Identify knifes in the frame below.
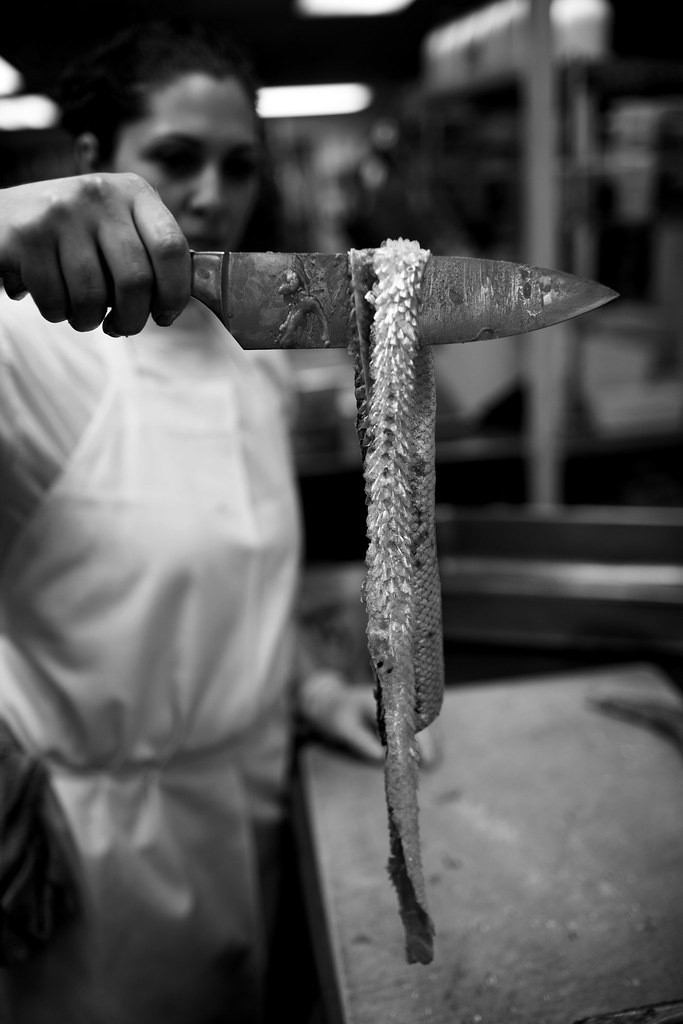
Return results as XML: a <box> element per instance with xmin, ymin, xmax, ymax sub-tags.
<box><xmin>1</xmin><ymin>249</ymin><xmax>622</xmax><ymax>350</ymax></box>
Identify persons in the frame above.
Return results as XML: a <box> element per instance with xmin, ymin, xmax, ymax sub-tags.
<box><xmin>0</xmin><ymin>28</ymin><xmax>443</xmax><ymax>1024</ymax></box>
